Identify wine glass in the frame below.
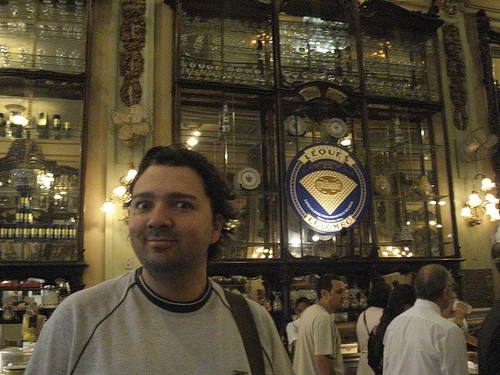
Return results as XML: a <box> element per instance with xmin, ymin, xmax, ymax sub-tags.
<box><xmin>180</xmin><ymin>60</ymin><xmax>423</xmax><ymax>95</ymax></box>
<box><xmin>0</xmin><ymin>0</ymin><xmax>84</xmax><ymax>72</ymax></box>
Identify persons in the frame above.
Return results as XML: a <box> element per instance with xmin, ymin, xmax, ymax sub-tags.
<box><xmin>491</xmin><ymin>226</ymin><xmax>500</xmax><ymax>273</ymax></box>
<box><xmin>286</xmin><ymin>296</ymin><xmax>310</xmax><ymax>356</ymax></box>
<box><xmin>353</xmin><ymin>282</ymin><xmax>393</xmax><ymax>375</ymax></box>
<box><xmin>291</xmin><ymin>274</ymin><xmax>346</xmax><ymax>375</ymax></box>
<box><xmin>365</xmin><ymin>283</ymin><xmax>417</xmax><ymax>375</ymax></box>
<box><xmin>438</xmin><ymin>283</ymin><xmax>469</xmax><ymax>334</ymax></box>
<box><xmin>23</xmin><ymin>145</ymin><xmax>296</xmax><ymax>375</ymax></box>
<box><xmin>382</xmin><ymin>264</ymin><xmax>469</xmax><ymax>375</ymax></box>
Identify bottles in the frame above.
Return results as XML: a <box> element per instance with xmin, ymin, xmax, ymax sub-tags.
<box><xmin>0</xmin><ymin>111</ymin><xmax>71</xmax><ymax>140</ymax></box>
<box><xmin>0</xmin><ymin>175</ymin><xmax>80</xmax><ymax>240</ymax></box>
<box><xmin>23</xmin><ymin>315</ymin><xmax>41</xmax><ymax>356</ymax></box>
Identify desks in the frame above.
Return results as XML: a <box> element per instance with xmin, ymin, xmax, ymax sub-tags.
<box><xmin>0</xmin><ymin>346</ymin><xmax>34</xmax><ymax>375</ymax></box>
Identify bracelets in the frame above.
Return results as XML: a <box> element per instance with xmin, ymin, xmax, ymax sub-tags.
<box><xmin>455</xmin><ymin>322</ymin><xmax>463</xmax><ymax>328</ymax></box>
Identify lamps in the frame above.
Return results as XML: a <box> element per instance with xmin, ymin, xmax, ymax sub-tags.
<box><xmin>461</xmin><ymin>173</ymin><xmax>500</xmax><ymax>228</ymax></box>
<box><xmin>100</xmin><ymin>163</ymin><xmax>137</xmax><ymax>227</ymax></box>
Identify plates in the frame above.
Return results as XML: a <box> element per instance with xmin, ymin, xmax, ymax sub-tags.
<box><xmin>3</xmin><ymin>362</ymin><xmax>28</xmax><ymax>372</ymax></box>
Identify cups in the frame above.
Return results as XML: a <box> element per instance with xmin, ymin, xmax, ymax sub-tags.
<box><xmin>453</xmin><ymin>301</ymin><xmax>472</xmax><ymax>314</ymax></box>
